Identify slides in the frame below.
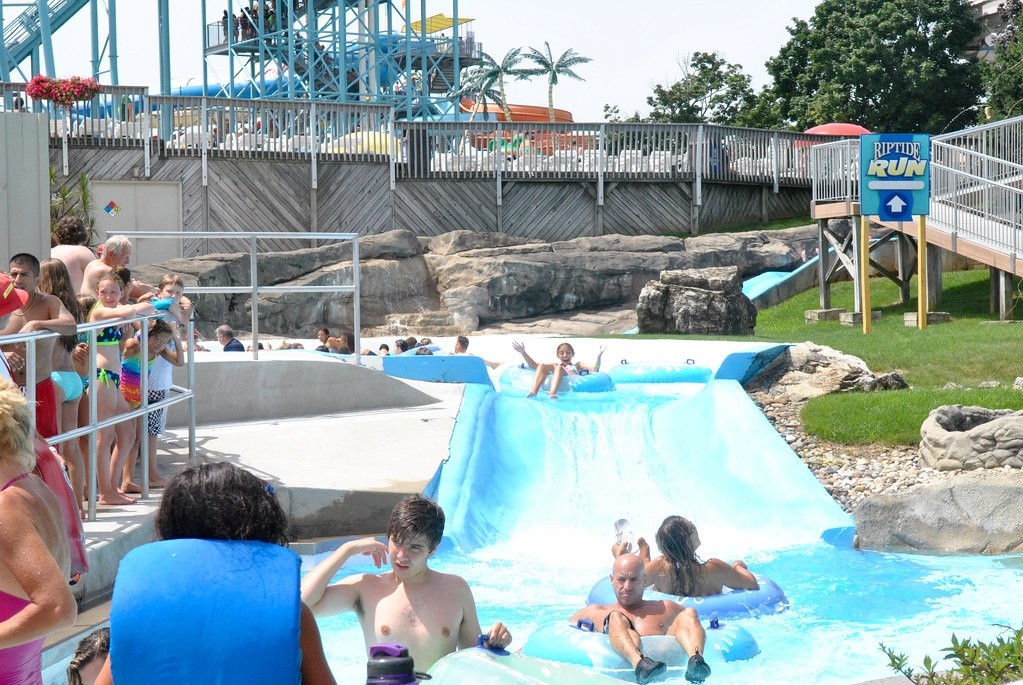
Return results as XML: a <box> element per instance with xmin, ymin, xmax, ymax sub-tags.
<box><xmin>423</xmin><ymin>378</ymin><xmax>862</xmax><ymax>554</ymax></box>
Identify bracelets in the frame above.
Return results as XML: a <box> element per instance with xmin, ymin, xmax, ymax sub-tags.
<box><xmin>734</xmin><ymin>564</ymin><xmax>744</xmax><ymax>567</ymax></box>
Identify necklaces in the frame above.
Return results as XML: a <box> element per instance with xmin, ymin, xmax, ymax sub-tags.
<box><xmin>13</xmin><ymin>291</ymin><xmax>37</xmax><ymax>316</ymax></box>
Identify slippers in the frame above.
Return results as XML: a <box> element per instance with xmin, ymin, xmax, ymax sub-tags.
<box><xmin>615</xmin><ymin>519</ymin><xmax>639</xmax><ymax>554</ymax></box>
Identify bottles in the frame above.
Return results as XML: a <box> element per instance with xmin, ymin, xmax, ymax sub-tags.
<box><xmin>366</xmin><ymin>643</ymin><xmax>418</xmax><ymax>685</ymax></box>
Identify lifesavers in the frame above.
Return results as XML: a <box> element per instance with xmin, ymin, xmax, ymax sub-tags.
<box><xmin>610</xmin><ymin>359</ymin><xmax>712</xmax><ymax>382</ymax></box>
<box><xmin>586</xmin><ymin>573</ymin><xmax>790</xmax><ymax>619</ymax></box>
<box><xmin>499</xmin><ymin>364</ymin><xmax>616</xmax><ymax>393</ymax></box>
<box><xmin>520</xmin><ymin>617</ymin><xmax>762</xmax><ymax>673</ymax></box>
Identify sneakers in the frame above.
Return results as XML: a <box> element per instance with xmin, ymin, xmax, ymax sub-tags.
<box><xmin>634</xmin><ymin>654</ymin><xmax>667</xmax><ymax>684</ymax></box>
<box><xmin>684</xmin><ymin>646</ymin><xmax>711</xmax><ymax>685</ymax></box>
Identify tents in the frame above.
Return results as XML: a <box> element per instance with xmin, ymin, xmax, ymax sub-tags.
<box><xmin>401</xmin><ymin>13</ymin><xmax>476</xmax><ymax>38</ymax></box>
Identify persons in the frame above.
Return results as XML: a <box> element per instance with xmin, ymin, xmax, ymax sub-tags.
<box><xmin>213</xmin><ymin>0</ymin><xmax>464</xmax><ymax>148</ymax></box>
<box><xmin>298</xmin><ymin>492</ymin><xmax>513</xmax><ymax>674</ymax></box>
<box><xmin>91</xmin><ymin>460</ymin><xmax>339</xmax><ymax>685</ymax></box>
<box><xmin>65</xmin><ymin>628</ymin><xmax>111</xmax><ymax>685</ymax></box>
<box><xmin>1</xmin><ymin>372</ymin><xmax>80</xmax><ymax>685</ymax></box>
<box><xmin>0</xmin><ymin>218</ymin><xmax>499</xmax><ymax>685</ymax></box>
<box><xmin>572</xmin><ymin>142</ymin><xmax>585</xmax><ymax>158</ymax></box>
<box><xmin>12</xmin><ymin>97</ymin><xmax>31</xmax><ymax>113</ymax></box>
<box><xmin>512</xmin><ymin>341</ymin><xmax>608</xmax><ymax>398</ymax></box>
<box><xmin>570</xmin><ymin>554</ymin><xmax>710</xmax><ymax>685</ymax></box>
<box><xmin>519</xmin><ymin>131</ymin><xmax>537</xmax><ymax>157</ymax></box>
<box><xmin>611</xmin><ymin>515</ymin><xmax>758</xmax><ymax>598</ymax></box>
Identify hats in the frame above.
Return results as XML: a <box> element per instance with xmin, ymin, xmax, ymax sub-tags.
<box><xmin>185</xmin><ymin>329</ymin><xmax>198</xmax><ymax>339</ymax></box>
